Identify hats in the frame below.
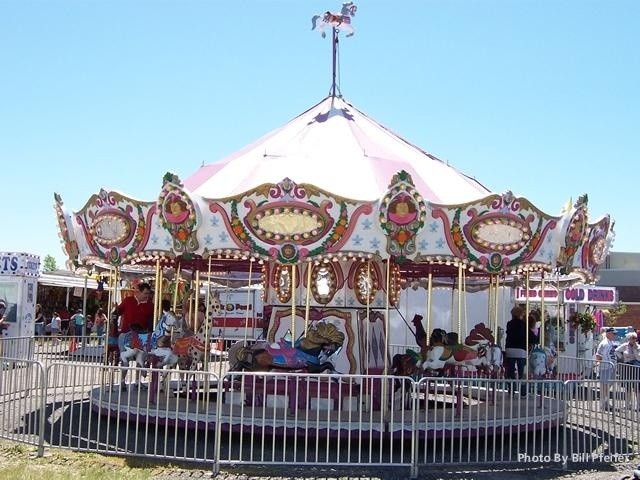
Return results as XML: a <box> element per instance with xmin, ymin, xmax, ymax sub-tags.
<box><xmin>606</xmin><ymin>327</ymin><xmax>619</xmax><ymax>335</ymax></box>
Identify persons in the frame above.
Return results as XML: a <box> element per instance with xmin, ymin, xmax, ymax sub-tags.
<box><xmin>505</xmin><ymin>306</ymin><xmax>551</xmax><ymax>399</ymax></box>
<box><xmin>35</xmin><ymin>304</ymin><xmax>108</xmax><ymax>346</ymax></box>
<box><xmin>0</xmin><ymin>298</ymin><xmax>10</xmax><ymax>357</ymax></box>
<box><xmin>596</xmin><ymin>328</ymin><xmax>618</xmax><ymax>411</ymax></box>
<box><xmin>114</xmin><ymin>282</ymin><xmax>155</xmax><ymax>391</ymax></box>
<box><xmin>615</xmin><ymin>330</ymin><xmax>640</xmax><ymax>411</ymax></box>
<box><xmin>198</xmin><ymin>303</ymin><xmax>207</xmax><ymax>313</ymax></box>
<box><xmin>185</xmin><ymin>300</ymin><xmax>204</xmax><ymax>331</ymax></box>
